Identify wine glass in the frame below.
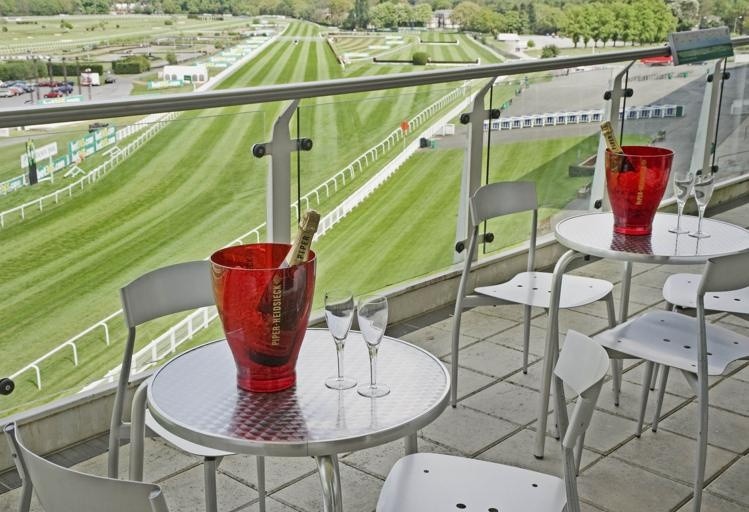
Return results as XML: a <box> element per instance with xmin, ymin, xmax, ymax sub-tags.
<box><xmin>355</xmin><ymin>295</ymin><xmax>391</xmax><ymax>399</ymax></box>
<box><xmin>319</xmin><ymin>289</ymin><xmax>358</xmax><ymax>389</ymax></box>
<box><xmin>668</xmin><ymin>171</ymin><xmax>694</xmax><ymax>234</ymax></box>
<box><xmin>688</xmin><ymin>174</ymin><xmax>715</xmax><ymax>238</ymax></box>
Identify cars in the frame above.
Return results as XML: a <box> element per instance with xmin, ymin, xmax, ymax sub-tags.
<box><xmin>0</xmin><ymin>76</ymin><xmax>75</xmax><ymax>100</ymax></box>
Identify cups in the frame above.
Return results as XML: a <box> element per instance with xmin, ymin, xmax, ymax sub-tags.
<box><xmin>227</xmin><ymin>386</ymin><xmax>316</xmax><ymax>445</ymax></box>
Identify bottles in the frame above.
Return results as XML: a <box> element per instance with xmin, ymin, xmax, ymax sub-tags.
<box><xmin>244</xmin><ymin>211</ymin><xmax>321</xmax><ymax>367</ymax></box>
<box><xmin>600</xmin><ymin>120</ymin><xmax>635</xmax><ymax>173</ymax></box>
